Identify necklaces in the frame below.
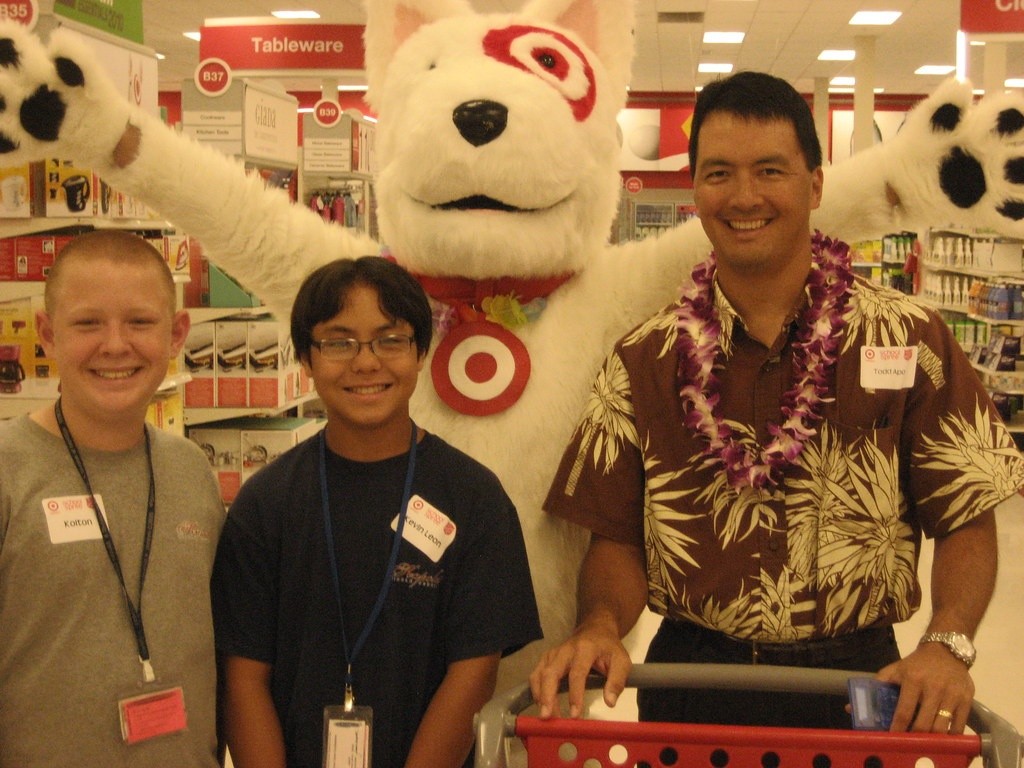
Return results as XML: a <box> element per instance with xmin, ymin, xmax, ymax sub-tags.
<box><xmin>676</xmin><ymin>227</ymin><xmax>854</xmax><ymax>495</ymax></box>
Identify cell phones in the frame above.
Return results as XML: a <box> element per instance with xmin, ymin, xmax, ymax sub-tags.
<box><xmin>847</xmin><ymin>676</ymin><xmax>921</xmax><ymax>733</ymax></box>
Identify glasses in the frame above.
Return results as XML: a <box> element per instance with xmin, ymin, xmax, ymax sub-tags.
<box><xmin>311</xmin><ymin>333</ymin><xmax>416</xmax><ymax>360</ymax></box>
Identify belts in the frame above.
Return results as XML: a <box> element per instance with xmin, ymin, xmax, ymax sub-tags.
<box><xmin>659</xmin><ymin>619</ymin><xmax>894</xmax><ymax>664</ymax></box>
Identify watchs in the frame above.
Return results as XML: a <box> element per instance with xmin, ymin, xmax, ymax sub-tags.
<box><xmin>919</xmin><ymin>632</ymin><xmax>977</xmax><ymax>671</ymax></box>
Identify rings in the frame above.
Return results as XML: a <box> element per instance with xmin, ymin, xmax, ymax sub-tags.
<box><xmin>937</xmin><ymin>710</ymin><xmax>953</xmax><ymax>720</ymax></box>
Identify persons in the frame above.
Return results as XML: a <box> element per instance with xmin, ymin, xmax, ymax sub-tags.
<box><xmin>0</xmin><ymin>230</ymin><xmax>229</xmax><ymax>768</ymax></box>
<box><xmin>214</xmin><ymin>255</ymin><xmax>519</xmax><ymax>768</ymax></box>
<box><xmin>532</xmin><ymin>73</ymin><xmax>999</xmax><ymax>735</ymax></box>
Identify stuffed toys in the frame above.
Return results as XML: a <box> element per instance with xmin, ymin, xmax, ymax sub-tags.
<box><xmin>0</xmin><ymin>0</ymin><xmax>1024</xmax><ymax>768</ymax></box>
